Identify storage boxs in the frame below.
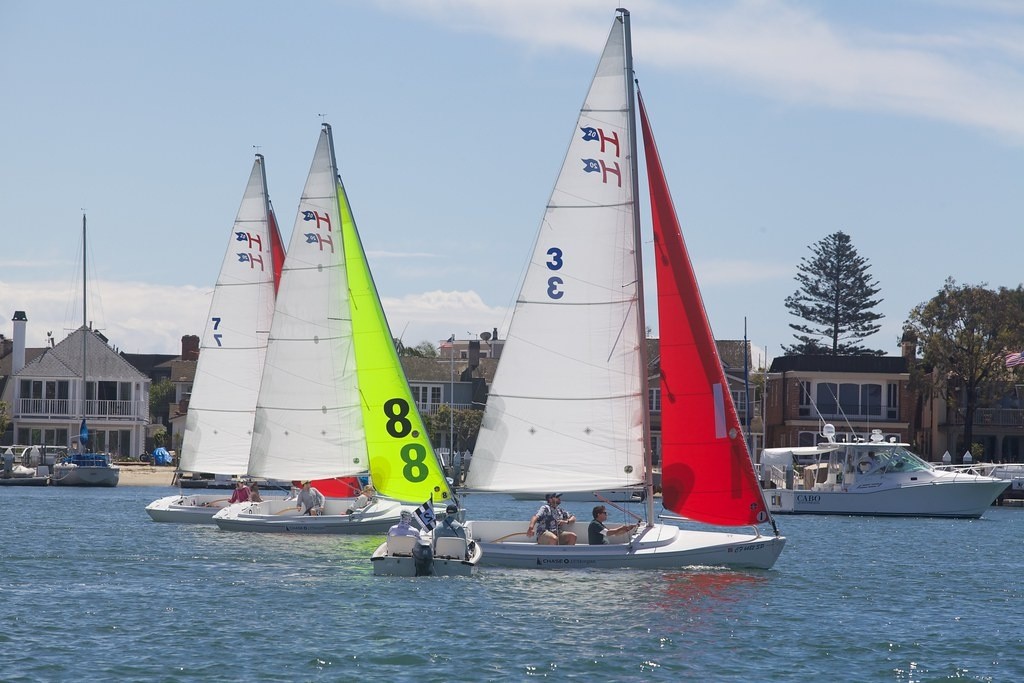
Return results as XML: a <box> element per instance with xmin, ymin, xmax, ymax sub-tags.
<box><xmin>803</xmin><ymin>463</ymin><xmax>839</xmax><ymax>490</ymax></box>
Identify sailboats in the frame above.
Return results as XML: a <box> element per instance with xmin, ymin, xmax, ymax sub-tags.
<box><xmin>52</xmin><ymin>214</ymin><xmax>119</xmax><ymax>487</ymax></box>
<box><xmin>335</xmin><ymin>174</ymin><xmax>484</xmax><ymax>577</ymax></box>
<box><xmin>145</xmin><ymin>154</ymin><xmax>372</xmax><ymax>525</ymax></box>
<box><xmin>461</xmin><ymin>6</ymin><xmax>788</xmax><ymax>570</ymax></box>
<box><xmin>212</xmin><ymin>123</ymin><xmax>467</xmax><ymax>534</ymax></box>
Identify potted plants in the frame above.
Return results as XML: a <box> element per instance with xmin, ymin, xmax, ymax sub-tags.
<box><xmin>149</xmin><ymin>377</ymin><xmax>176</xmax><ymax>423</ymax></box>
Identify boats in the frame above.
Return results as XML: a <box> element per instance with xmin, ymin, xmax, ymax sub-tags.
<box><xmin>761</xmin><ymin>424</ymin><xmax>1024</xmax><ymax>520</ymax></box>
<box><xmin>512</xmin><ymin>485</ymin><xmax>645</xmax><ymax>503</ymax></box>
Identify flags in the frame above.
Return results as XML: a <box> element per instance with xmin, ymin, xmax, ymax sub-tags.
<box><xmin>1005</xmin><ymin>351</ymin><xmax>1024</xmax><ymax>367</ymax></box>
<box><xmin>414</xmin><ymin>499</ymin><xmax>436</xmax><ymax>533</ymax></box>
<box><xmin>438</xmin><ymin>337</ymin><xmax>455</xmax><ymax>348</ymax></box>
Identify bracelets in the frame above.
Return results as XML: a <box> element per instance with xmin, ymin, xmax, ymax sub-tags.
<box><xmin>567</xmin><ymin>520</ymin><xmax>569</xmax><ymax>524</ymax></box>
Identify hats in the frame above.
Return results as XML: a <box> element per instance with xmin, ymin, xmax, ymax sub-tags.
<box><xmin>447</xmin><ymin>505</ymin><xmax>458</xmax><ymax>513</ymax></box>
<box><xmin>238</xmin><ymin>479</ymin><xmax>244</xmax><ymax>483</ymax></box>
<box><xmin>301</xmin><ymin>480</ymin><xmax>312</xmax><ymax>485</ymax></box>
<box><xmin>545</xmin><ymin>493</ymin><xmax>563</xmax><ymax>500</ymax></box>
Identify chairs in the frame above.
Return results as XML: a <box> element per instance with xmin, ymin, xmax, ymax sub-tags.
<box><xmin>388</xmin><ymin>535</ymin><xmax>417</xmax><ymax>557</ymax></box>
<box><xmin>436</xmin><ymin>536</ymin><xmax>465</xmax><ymax>560</ymax></box>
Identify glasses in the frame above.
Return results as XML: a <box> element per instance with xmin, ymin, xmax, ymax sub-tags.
<box><xmin>602</xmin><ymin>511</ymin><xmax>606</xmax><ymax>514</ymax></box>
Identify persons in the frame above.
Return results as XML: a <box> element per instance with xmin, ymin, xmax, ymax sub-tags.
<box><xmin>251</xmin><ymin>481</ymin><xmax>258</xmax><ymax>492</ymax></box>
<box><xmin>433</xmin><ymin>504</ymin><xmax>468</xmax><ymax>555</ymax></box>
<box><xmin>869</xmin><ymin>451</ymin><xmax>875</xmax><ymax>458</ymax></box>
<box><xmin>228</xmin><ymin>478</ymin><xmax>251</xmax><ymax>503</ymax></box>
<box><xmin>347</xmin><ymin>485</ymin><xmax>374</xmax><ymax>514</ymax></box>
<box><xmin>387</xmin><ymin>510</ymin><xmax>421</xmax><ymax>552</ymax></box>
<box><xmin>526</xmin><ymin>492</ymin><xmax>577</xmax><ymax>545</ymax></box>
<box><xmin>588</xmin><ymin>505</ymin><xmax>636</xmax><ymax>545</ymax></box>
<box><xmin>297</xmin><ymin>479</ymin><xmax>325</xmax><ymax>516</ymax></box>
